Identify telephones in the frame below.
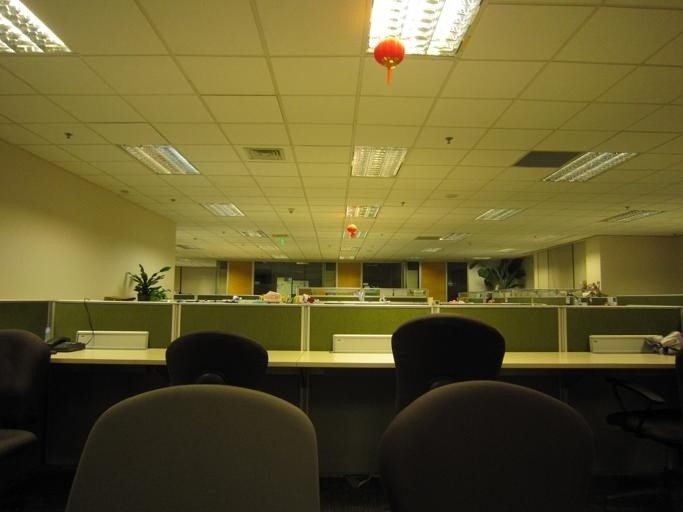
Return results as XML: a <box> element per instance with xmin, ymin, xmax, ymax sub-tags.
<box><xmin>45</xmin><ymin>336</ymin><xmax>86</xmax><ymax>352</ymax></box>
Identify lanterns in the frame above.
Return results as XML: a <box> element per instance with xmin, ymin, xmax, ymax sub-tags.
<box><xmin>372</xmin><ymin>34</ymin><xmax>406</xmax><ymax>85</ymax></box>
<box><xmin>347</xmin><ymin>224</ymin><xmax>357</xmax><ymax>239</ymax></box>
